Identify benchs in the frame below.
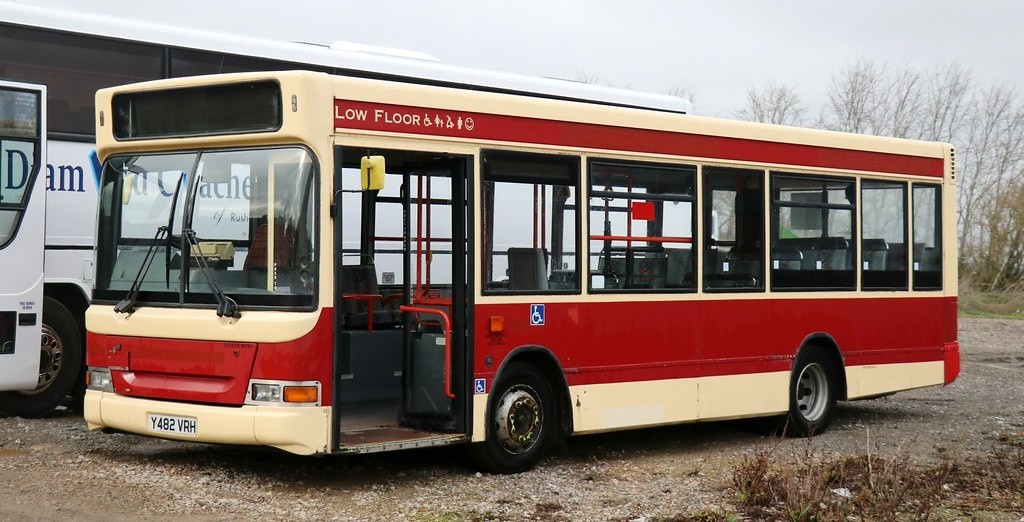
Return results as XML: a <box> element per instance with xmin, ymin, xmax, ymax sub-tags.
<box><xmin>597</xmin><ymin>237</ymin><xmax>925</xmax><ymax>288</ymax></box>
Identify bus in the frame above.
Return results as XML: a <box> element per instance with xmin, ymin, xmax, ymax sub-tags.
<box><xmin>81</xmin><ymin>69</ymin><xmax>964</xmax><ymax>474</ymax></box>
<box><xmin>0</xmin><ymin>79</ymin><xmax>725</xmax><ymax>422</ymax></box>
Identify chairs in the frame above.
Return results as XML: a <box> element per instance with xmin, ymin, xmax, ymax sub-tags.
<box><xmin>342</xmin><ymin>255</ymin><xmax>403</xmax><ymax>330</ymax></box>
<box><xmin>508</xmin><ymin>248</ymin><xmax>549</xmax><ymax>290</ymax></box>
